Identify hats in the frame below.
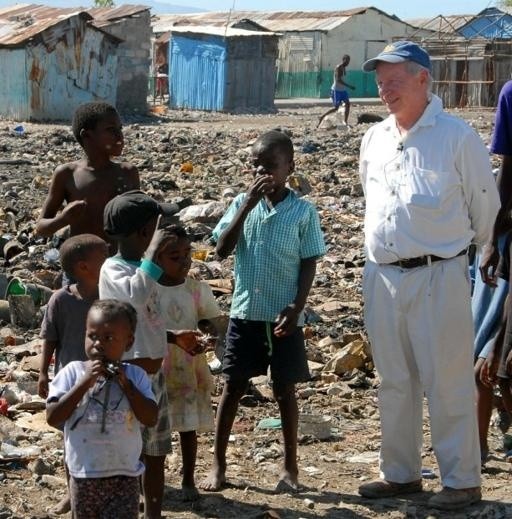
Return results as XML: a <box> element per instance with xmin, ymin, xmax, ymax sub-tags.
<box><xmin>362</xmin><ymin>41</ymin><xmax>432</xmax><ymax>72</ymax></box>
<box><xmin>104</xmin><ymin>190</ymin><xmax>179</xmax><ymax>237</ymax></box>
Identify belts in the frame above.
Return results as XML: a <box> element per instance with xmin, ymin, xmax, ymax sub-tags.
<box><xmin>388</xmin><ymin>249</ymin><xmax>467</xmax><ymax>268</ymax></box>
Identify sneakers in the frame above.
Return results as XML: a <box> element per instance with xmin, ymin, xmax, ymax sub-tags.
<box><xmin>427</xmin><ymin>488</ymin><xmax>481</xmax><ymax>509</ymax></box>
<box><xmin>359</xmin><ymin>479</ymin><xmax>422</xmax><ymax>498</ymax></box>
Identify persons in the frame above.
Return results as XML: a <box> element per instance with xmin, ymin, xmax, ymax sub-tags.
<box><xmin>194</xmin><ymin>131</ymin><xmax>326</xmax><ymax>492</ymax></box>
<box><xmin>46</xmin><ymin>300</ymin><xmax>158</xmax><ymax>519</ymax></box>
<box><xmin>35</xmin><ymin>103</ymin><xmax>143</xmax><ymax>257</ymax></box>
<box><xmin>97</xmin><ymin>189</ymin><xmax>214</xmax><ymax>519</ymax></box>
<box><xmin>152</xmin><ymin>224</ymin><xmax>222</xmax><ymax>501</ymax></box>
<box><xmin>38</xmin><ymin>234</ymin><xmax>109</xmax><ymax>514</ymax></box>
<box><xmin>316</xmin><ymin>54</ymin><xmax>356</xmax><ymax>130</ymax></box>
<box><xmin>472</xmin><ymin>82</ymin><xmax>512</xmax><ymax>477</ymax></box>
<box><xmin>359</xmin><ymin>40</ymin><xmax>502</xmax><ymax>512</ymax></box>
